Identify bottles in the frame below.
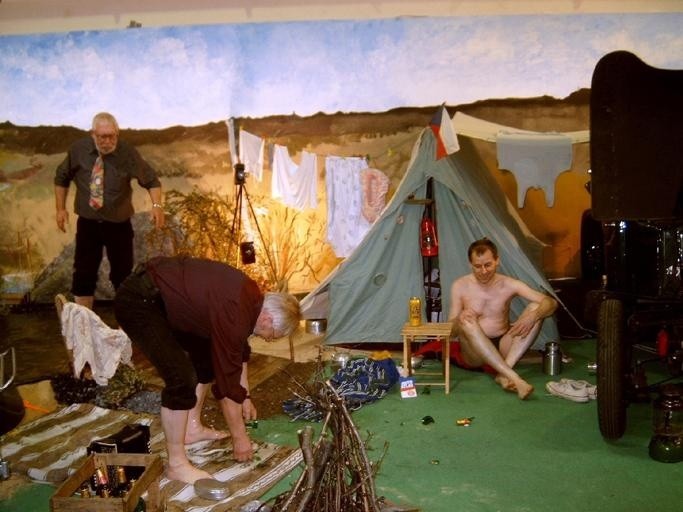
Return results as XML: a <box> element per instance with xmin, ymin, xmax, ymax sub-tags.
<box><xmin>586</xmin><ymin>362</ymin><xmax>596</xmax><ymax>375</ymax></box>
<box><xmin>79</xmin><ymin>466</ymin><xmax>145</xmax><ymax>512</ymax></box>
<box><xmin>408</xmin><ymin>296</ymin><xmax>421</xmax><ymax>326</ymax></box>
<box><xmin>542</xmin><ymin>342</ymin><xmax>561</xmax><ymax>376</ymax></box>
<box><xmin>599</xmin><ymin>275</ymin><xmax>608</xmax><ymax>299</ymax></box>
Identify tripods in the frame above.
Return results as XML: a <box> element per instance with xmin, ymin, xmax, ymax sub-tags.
<box><xmin>225</xmin><ymin>184</ymin><xmax>284</xmax><ymax>290</ymax></box>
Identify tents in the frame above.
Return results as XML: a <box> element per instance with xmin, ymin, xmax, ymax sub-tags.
<box><xmin>299</xmin><ymin>126</ymin><xmax>597</xmax><ymax>350</ymax></box>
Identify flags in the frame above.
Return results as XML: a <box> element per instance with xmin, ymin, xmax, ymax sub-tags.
<box><xmin>427</xmin><ymin>103</ymin><xmax>461</xmax><ymax>161</ymax></box>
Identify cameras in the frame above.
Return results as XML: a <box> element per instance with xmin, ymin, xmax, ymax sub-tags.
<box><xmin>234</xmin><ymin>163</ymin><xmax>246</xmax><ymax>185</ymax></box>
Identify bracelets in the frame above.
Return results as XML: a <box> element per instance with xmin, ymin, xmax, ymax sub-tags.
<box><xmin>152</xmin><ymin>203</ymin><xmax>162</xmax><ymax>209</ymax></box>
<box><xmin>244</xmin><ymin>396</ymin><xmax>250</xmax><ymax>399</ymax></box>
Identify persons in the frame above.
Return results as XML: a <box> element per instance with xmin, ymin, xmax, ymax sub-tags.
<box><xmin>53</xmin><ymin>112</ymin><xmax>166</xmax><ymax>311</ymax></box>
<box><xmin>447</xmin><ymin>240</ymin><xmax>558</xmax><ymax>399</ymax></box>
<box><xmin>113</xmin><ymin>256</ymin><xmax>301</xmax><ymax>487</ymax></box>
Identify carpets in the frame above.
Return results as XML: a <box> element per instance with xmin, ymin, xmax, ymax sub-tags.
<box><xmin>1</xmin><ymin>400</ymin><xmax>324</xmax><ymax>511</ymax></box>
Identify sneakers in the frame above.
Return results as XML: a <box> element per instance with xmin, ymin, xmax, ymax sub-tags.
<box><xmin>560</xmin><ymin>378</ymin><xmax>598</xmax><ymax>400</ymax></box>
<box><xmin>545</xmin><ymin>380</ymin><xmax>589</xmax><ymax>402</ymax></box>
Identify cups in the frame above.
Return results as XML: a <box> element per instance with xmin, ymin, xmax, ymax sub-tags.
<box><xmin>239</xmin><ymin>241</ymin><xmax>255</xmax><ymax>265</ymax></box>
<box><xmin>306</xmin><ymin>319</ymin><xmax>326</xmax><ymax>334</ymax></box>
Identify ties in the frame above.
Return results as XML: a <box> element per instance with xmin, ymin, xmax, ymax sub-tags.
<box><xmin>88</xmin><ymin>152</ymin><xmax>104</xmax><ymax>212</ymax></box>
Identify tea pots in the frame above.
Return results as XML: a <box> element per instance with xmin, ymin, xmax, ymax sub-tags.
<box><xmin>329</xmin><ymin>345</ymin><xmax>351</xmax><ymax>371</ymax></box>
<box><xmin>400</xmin><ymin>354</ymin><xmax>424</xmax><ymax>369</ymax></box>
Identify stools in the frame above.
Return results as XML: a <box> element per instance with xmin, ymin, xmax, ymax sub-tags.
<box><xmin>397</xmin><ymin>317</ymin><xmax>453</xmax><ymax>396</ymax></box>
